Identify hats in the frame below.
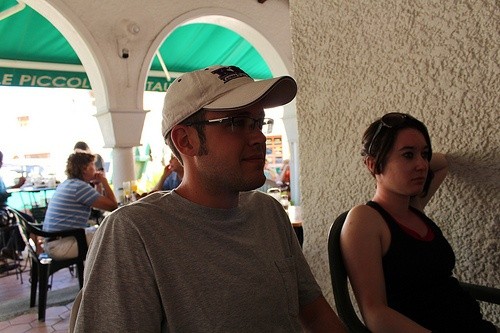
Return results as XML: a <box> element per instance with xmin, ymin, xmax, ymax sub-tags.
<box><xmin>162</xmin><ymin>66</ymin><xmax>297</xmax><ymax>140</ymax></box>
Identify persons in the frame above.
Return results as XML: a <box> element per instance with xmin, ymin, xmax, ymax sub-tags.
<box><xmin>41</xmin><ymin>151</ymin><xmax>118</xmax><ymax>261</ymax></box>
<box><xmin>68</xmin><ymin>64</ymin><xmax>349</xmax><ymax>333</ymax></box>
<box><xmin>151</xmin><ymin>150</ymin><xmax>185</xmax><ymax>195</ymax></box>
<box><xmin>0</xmin><ymin>150</ymin><xmax>46</xmax><ymax>261</ymax></box>
<box><xmin>74</xmin><ymin>140</ymin><xmax>106</xmax><ymax>228</ymax></box>
<box><xmin>340</xmin><ymin>111</ymin><xmax>499</xmax><ymax>332</ymax></box>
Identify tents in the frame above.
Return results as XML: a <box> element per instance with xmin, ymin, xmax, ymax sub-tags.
<box><xmin>0</xmin><ymin>0</ymin><xmax>280</xmax><ymax>94</ymax></box>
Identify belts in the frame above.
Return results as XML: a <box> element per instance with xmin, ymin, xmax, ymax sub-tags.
<box><xmin>46</xmin><ymin>236</ymin><xmax>67</xmax><ymax>243</ymax></box>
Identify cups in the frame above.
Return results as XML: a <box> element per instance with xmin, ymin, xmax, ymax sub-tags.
<box><xmin>288</xmin><ymin>206</ymin><xmax>295</xmax><ymax>221</ymax></box>
<box><xmin>48</xmin><ymin>174</ymin><xmax>56</xmax><ymax>186</ymax></box>
<box><xmin>132</xmin><ymin>181</ymin><xmax>138</xmax><ymax>192</ymax></box>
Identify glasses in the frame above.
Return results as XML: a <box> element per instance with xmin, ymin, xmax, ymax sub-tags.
<box><xmin>368</xmin><ymin>112</ymin><xmax>407</xmax><ymax>155</ymax></box>
<box><xmin>177</xmin><ymin>114</ymin><xmax>274</xmax><ymax>136</ymax></box>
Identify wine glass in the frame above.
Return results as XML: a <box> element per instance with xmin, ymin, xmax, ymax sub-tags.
<box><xmin>123</xmin><ymin>182</ymin><xmax>131</xmax><ymax>205</ymax></box>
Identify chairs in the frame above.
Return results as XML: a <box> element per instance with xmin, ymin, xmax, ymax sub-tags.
<box><xmin>0</xmin><ymin>207</ymin><xmax>89</xmax><ymax>321</ymax></box>
<box><xmin>328</xmin><ymin>210</ymin><xmax>500</xmax><ymax>333</ymax></box>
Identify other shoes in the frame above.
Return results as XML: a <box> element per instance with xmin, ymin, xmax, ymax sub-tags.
<box><xmin>0</xmin><ymin>247</ymin><xmax>24</xmax><ymax>261</ymax></box>
<box><xmin>0</xmin><ymin>263</ymin><xmax>20</xmax><ymax>274</ymax></box>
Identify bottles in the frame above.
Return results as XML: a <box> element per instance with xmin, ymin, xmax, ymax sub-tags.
<box><xmin>280</xmin><ymin>195</ymin><xmax>289</xmax><ymax>211</ymax></box>
